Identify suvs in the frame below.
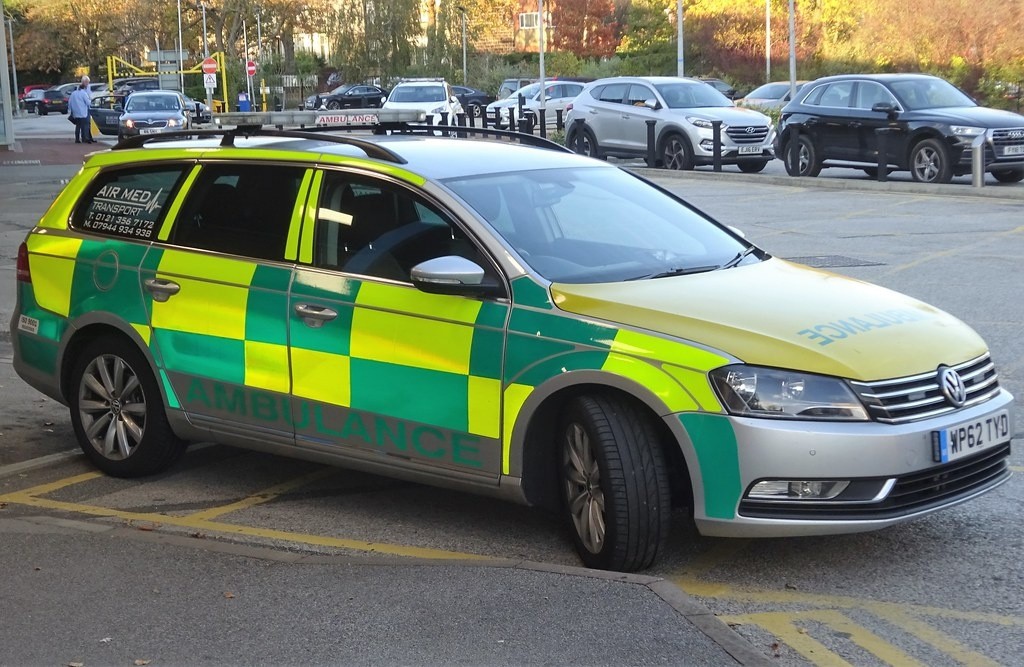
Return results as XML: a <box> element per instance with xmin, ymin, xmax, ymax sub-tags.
<box><xmin>9</xmin><ymin>126</ymin><xmax>1016</xmax><ymax>575</ymax></box>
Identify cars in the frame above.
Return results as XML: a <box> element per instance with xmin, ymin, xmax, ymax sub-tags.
<box><xmin>177</xmin><ymin>90</ymin><xmax>212</xmax><ymax>124</ymax></box>
<box><xmin>774</xmin><ymin>74</ymin><xmax>1024</xmax><ymax>183</ymax></box>
<box><xmin>114</xmin><ymin>89</ymin><xmax>191</xmax><ymax>144</ymax></box>
<box><xmin>47</xmin><ymin>82</ymin><xmax>106</xmax><ymax>99</ymax></box>
<box><xmin>95</xmin><ymin>76</ymin><xmax>160</xmax><ymax>91</ymax></box>
<box><xmin>563</xmin><ymin>76</ymin><xmax>778</xmax><ymax>174</ymax></box>
<box><xmin>87</xmin><ymin>97</ymin><xmax>125</xmax><ymax>132</ymax></box>
<box><xmin>698</xmin><ymin>77</ymin><xmax>745</xmax><ymax>101</ymax></box>
<box><xmin>304</xmin><ymin>84</ymin><xmax>390</xmax><ymax>110</ymax></box>
<box><xmin>18</xmin><ymin>88</ymin><xmax>69</xmax><ymax>115</ymax></box>
<box><xmin>451</xmin><ymin>86</ymin><xmax>496</xmax><ymax>118</ymax></box>
<box><xmin>19</xmin><ymin>85</ymin><xmax>52</xmax><ymax>99</ymax></box>
<box><xmin>734</xmin><ymin>81</ymin><xmax>814</xmax><ymax>117</ymax></box>
<box><xmin>485</xmin><ymin>81</ymin><xmax>588</xmax><ymax>129</ymax></box>
<box><xmin>378</xmin><ymin>77</ymin><xmax>465</xmax><ymax>141</ymax></box>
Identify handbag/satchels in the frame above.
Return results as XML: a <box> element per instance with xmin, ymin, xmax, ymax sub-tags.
<box><xmin>68</xmin><ymin>115</ymin><xmax>80</xmax><ymax>125</ymax></box>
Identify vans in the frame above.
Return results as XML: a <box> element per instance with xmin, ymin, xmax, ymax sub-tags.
<box><xmin>495</xmin><ymin>78</ymin><xmax>539</xmax><ymax>100</ymax></box>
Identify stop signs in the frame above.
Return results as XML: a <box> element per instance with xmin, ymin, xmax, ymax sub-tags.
<box><xmin>246</xmin><ymin>62</ymin><xmax>256</xmax><ymax>77</ymax></box>
<box><xmin>202</xmin><ymin>58</ymin><xmax>217</xmax><ymax>74</ymax></box>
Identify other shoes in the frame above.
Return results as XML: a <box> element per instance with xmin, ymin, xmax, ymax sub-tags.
<box><xmin>75</xmin><ymin>140</ymin><xmax>81</xmax><ymax>143</ymax></box>
<box><xmin>85</xmin><ymin>141</ymin><xmax>91</xmax><ymax>144</ymax></box>
<box><xmin>91</xmin><ymin>138</ymin><xmax>97</xmax><ymax>142</ymax></box>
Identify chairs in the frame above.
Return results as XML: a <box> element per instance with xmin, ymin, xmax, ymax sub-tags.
<box><xmin>344</xmin><ymin>196</ymin><xmax>424</xmax><ymax>281</ymax></box>
<box><xmin>825</xmin><ymin>91</ymin><xmax>841</xmax><ymax>106</ymax></box>
<box><xmin>861</xmin><ymin>85</ymin><xmax>878</xmax><ymax>109</ymax></box>
<box><xmin>666</xmin><ymin>89</ymin><xmax>680</xmax><ymax>105</ymax></box>
<box><xmin>445</xmin><ymin>182</ymin><xmax>524</xmax><ymax>273</ymax></box>
<box><xmin>889</xmin><ymin>90</ymin><xmax>908</xmax><ymax>109</ymax></box>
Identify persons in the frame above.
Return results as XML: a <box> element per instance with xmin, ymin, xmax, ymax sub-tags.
<box><xmin>69</xmin><ymin>85</ymin><xmax>91</xmax><ymax>143</ymax></box>
<box><xmin>122</xmin><ymin>89</ymin><xmax>130</xmax><ymax>103</ymax></box>
<box><xmin>315</xmin><ymin>95</ymin><xmax>322</xmax><ymax>109</ymax></box>
<box><xmin>77</xmin><ymin>76</ymin><xmax>97</xmax><ymax>142</ymax></box>
